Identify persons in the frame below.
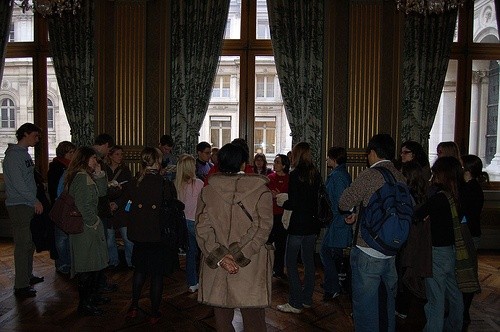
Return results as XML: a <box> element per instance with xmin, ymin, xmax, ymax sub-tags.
<box><xmin>460</xmin><ymin>154</ymin><xmax>483</xmax><ymax>332</ymax></box>
<box><xmin>203</xmin><ymin>139</ymin><xmax>253</xmax><ymax>188</ymax></box>
<box><xmin>319</xmin><ymin>146</ymin><xmax>352</xmax><ymax>301</ymax></box>
<box><xmin>64</xmin><ymin>147</ymin><xmax>110</xmax><ymax>318</ymax></box>
<box><xmin>95</xmin><ymin>135</ymin><xmax>114</xmax><ymax>155</ymax></box>
<box><xmin>337</xmin><ymin>134</ymin><xmax>412</xmax><ymax>332</ymax></box>
<box><xmin>48</xmin><ymin>141</ymin><xmax>76</xmax><ymax>276</ymax></box>
<box><xmin>113</xmin><ymin>146</ymin><xmax>177</xmax><ymax>324</ymax></box>
<box><xmin>264</xmin><ymin>154</ymin><xmax>301</xmax><ymax>262</ymax></box>
<box><xmin>412</xmin><ymin>155</ymin><xmax>475</xmax><ymax>332</ymax></box>
<box><xmin>394</xmin><ymin>141</ymin><xmax>432</xmax><ymax>319</ymax></box>
<box><xmin>437</xmin><ymin>142</ymin><xmax>461</xmax><ymax>160</ymax></box>
<box><xmin>173</xmin><ymin>154</ymin><xmax>205</xmax><ymax>293</ymax></box>
<box><xmin>3</xmin><ymin>122</ymin><xmax>45</xmax><ymax>297</ymax></box>
<box><xmin>194</xmin><ymin>143</ymin><xmax>274</xmax><ymax>332</ymax></box>
<box><xmin>98</xmin><ymin>146</ymin><xmax>134</xmax><ymax>270</ymax></box>
<box><xmin>254</xmin><ymin>153</ymin><xmax>273</xmax><ymax>176</ymax></box>
<box><xmin>287</xmin><ymin>151</ymin><xmax>294</xmax><ymax>169</ymax></box>
<box><xmin>276</xmin><ymin>142</ymin><xmax>327</xmax><ymax>314</ymax></box>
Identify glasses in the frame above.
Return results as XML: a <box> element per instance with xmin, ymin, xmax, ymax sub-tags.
<box><xmin>400</xmin><ymin>151</ymin><xmax>412</xmax><ymax>155</ymax></box>
<box><xmin>437</xmin><ymin>150</ymin><xmax>443</xmax><ymax>154</ymax></box>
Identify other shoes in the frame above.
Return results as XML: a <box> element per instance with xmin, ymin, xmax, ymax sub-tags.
<box><xmin>14</xmin><ymin>287</ymin><xmax>36</xmax><ymax>294</ymax></box>
<box><xmin>319</xmin><ymin>282</ymin><xmax>343</xmax><ymax>302</ymax></box>
<box><xmin>178</xmin><ymin>248</ymin><xmax>187</xmax><ymax>255</ymax></box>
<box><xmin>77</xmin><ymin>303</ymin><xmax>103</xmax><ymax>315</ymax></box>
<box><xmin>91</xmin><ymin>295</ymin><xmax>113</xmax><ymax>305</ymax></box>
<box><xmin>56</xmin><ymin>265</ymin><xmax>71</xmax><ymax>273</ymax></box>
<box><xmin>189</xmin><ymin>282</ymin><xmax>199</xmax><ymax>292</ymax></box>
<box><xmin>127</xmin><ymin>308</ymin><xmax>137</xmax><ymax>317</ymax></box>
<box><xmin>150</xmin><ymin>316</ymin><xmax>161</xmax><ymax>324</ymax></box>
<box><xmin>30</xmin><ymin>275</ymin><xmax>44</xmax><ymax>282</ymax></box>
<box><xmin>97</xmin><ymin>284</ymin><xmax>117</xmax><ymax>293</ymax></box>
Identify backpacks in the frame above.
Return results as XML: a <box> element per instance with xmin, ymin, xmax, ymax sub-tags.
<box><xmin>353</xmin><ymin>166</ymin><xmax>414</xmax><ymax>256</ymax></box>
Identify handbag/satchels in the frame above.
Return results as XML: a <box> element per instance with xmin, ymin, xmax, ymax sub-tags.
<box><xmin>48</xmin><ymin>170</ymin><xmax>84</xmax><ymax>234</ymax></box>
<box><xmin>159</xmin><ymin>180</ymin><xmax>188</xmax><ymax>249</ymax></box>
<box><xmin>312</xmin><ymin>181</ymin><xmax>334</xmax><ymax>229</ymax></box>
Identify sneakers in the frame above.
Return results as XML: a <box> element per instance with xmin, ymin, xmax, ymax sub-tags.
<box><xmin>276</xmin><ymin>303</ymin><xmax>311</xmax><ymax>313</ymax></box>
